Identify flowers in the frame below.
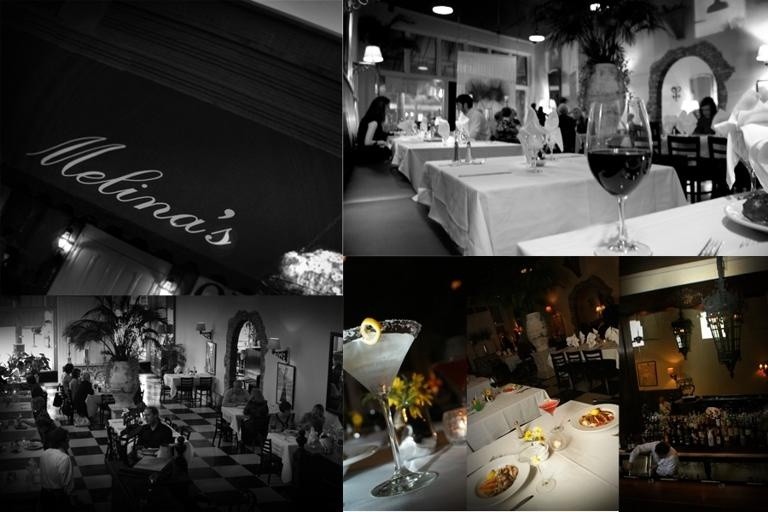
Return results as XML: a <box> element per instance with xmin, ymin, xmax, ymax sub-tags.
<box><xmin>361</xmin><ymin>372</ymin><xmax>444</xmax><ymax>422</ymax></box>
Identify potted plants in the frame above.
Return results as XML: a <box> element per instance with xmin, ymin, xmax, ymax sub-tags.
<box><xmin>530</xmin><ymin>0</ymin><xmax>682</xmax><ymax>154</ymax></box>
<box><xmin>61</xmin><ymin>295</ymin><xmax>172</xmax><ymax>406</ymax></box>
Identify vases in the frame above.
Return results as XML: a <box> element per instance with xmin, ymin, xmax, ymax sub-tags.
<box><xmin>407</xmin><ymin>405</ymin><xmax>441</xmax><ymax>450</ymax></box>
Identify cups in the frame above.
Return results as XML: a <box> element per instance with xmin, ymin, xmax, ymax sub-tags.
<box><xmin>549</xmin><ymin>434</ymin><xmax>566</xmax><ymax>450</ymax></box>
<box><xmin>442</xmin><ymin>407</ymin><xmax>467</xmax><ymax>444</ymax></box>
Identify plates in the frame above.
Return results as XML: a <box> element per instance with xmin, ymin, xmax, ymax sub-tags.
<box><xmin>722</xmin><ymin>194</ymin><xmax>768</xmax><ymax>232</ymax></box>
<box><xmin>502</xmin><ymin>383</ymin><xmax>521</xmax><ymax>395</ymax></box>
<box><xmin>569</xmin><ymin>403</ymin><xmax>618</xmax><ymax>433</ymax></box>
<box><xmin>343</xmin><ymin>444</ymin><xmax>376</xmax><ymax>467</ymax></box>
<box><xmin>466</xmin><ymin>453</ymin><xmax>529</xmax><ymax>509</ymax></box>
<box><xmin>23</xmin><ymin>442</ymin><xmax>43</xmax><ymax>450</ymax></box>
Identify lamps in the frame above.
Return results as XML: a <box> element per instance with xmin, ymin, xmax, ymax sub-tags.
<box><xmin>595</xmin><ymin>305</ymin><xmax>605</xmax><ymax>319</ymax></box>
<box><xmin>352</xmin><ymin>44</ymin><xmax>385</xmax><ymax>74</ymax></box>
<box><xmin>194</xmin><ymin>322</ymin><xmax>212</xmax><ymax>341</ymax></box>
<box><xmin>280</xmin><ymin>248</ymin><xmax>343</xmax><ymax>296</ymax></box>
<box><xmin>670</xmin><ymin>308</ymin><xmax>692</xmax><ymax>361</ymax></box>
<box><xmin>157</xmin><ymin>274</ymin><xmax>177</xmax><ymax>295</ymax></box>
<box><xmin>667</xmin><ymin>368</ymin><xmax>677</xmax><ymax>382</ymax></box>
<box><xmin>267</xmin><ymin>337</ymin><xmax>289</xmax><ymax>362</ymax></box>
<box><xmin>524</xmin><ymin>0</ymin><xmax>545</xmax><ymax>44</ymax></box>
<box><xmin>705</xmin><ymin>257</ymin><xmax>747</xmax><ymax>379</ymax></box>
<box><xmin>57</xmin><ymin>228</ymin><xmax>74</xmax><ymax>254</ymax></box>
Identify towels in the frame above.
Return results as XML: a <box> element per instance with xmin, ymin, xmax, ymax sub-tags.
<box><xmin>565</xmin><ymin>326</ymin><xmax>619</xmax><ymax>348</ymax></box>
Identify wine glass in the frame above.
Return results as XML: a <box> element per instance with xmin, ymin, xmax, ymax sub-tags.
<box><xmin>516</xmin><ymin>434</ymin><xmax>557</xmax><ymax>494</ymax></box>
<box><xmin>537</xmin><ymin>398</ymin><xmax>566</xmax><ymax>434</ymax></box>
<box><xmin>522</xmin><ymin>127</ymin><xmax>560</xmax><ymax>175</ymax></box>
<box><xmin>343</xmin><ymin>315</ymin><xmax>441</xmax><ymax>499</ymax></box>
<box><xmin>585</xmin><ymin>93</ymin><xmax>659</xmax><ymax>256</ymax></box>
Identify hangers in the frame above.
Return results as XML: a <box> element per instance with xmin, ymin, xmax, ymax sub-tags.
<box><xmin>343</xmin><ymin>420</ymin><xmax>467</xmax><ymax>511</ymax></box>
<box><xmin>466</xmin><ymin>336</ymin><xmax>619</xmax><ymax>511</ymax></box>
<box><xmin>386</xmin><ymin>123</ymin><xmax>767</xmax><ymax>256</ymax></box>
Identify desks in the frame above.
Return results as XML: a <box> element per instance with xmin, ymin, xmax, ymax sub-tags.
<box><xmin>620</xmin><ymin>478</ymin><xmax>768</xmax><ymax>512</ymax></box>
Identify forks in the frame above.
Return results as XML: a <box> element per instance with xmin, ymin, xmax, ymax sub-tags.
<box><xmin>697</xmin><ymin>236</ymin><xmax>725</xmax><ymax>257</ymax></box>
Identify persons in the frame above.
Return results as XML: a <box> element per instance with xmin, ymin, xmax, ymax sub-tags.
<box><xmin>692</xmin><ymin>96</ymin><xmax>719</xmax><ymax>133</ymax></box>
<box><xmin>624</xmin><ymin>439</ymin><xmax>681</xmax><ymax>476</ymax></box>
<box><xmin>453</xmin><ymin>96</ymin><xmax>492</xmax><ymax>140</ymax></box>
<box><xmin>136</xmin><ymin>405</ymin><xmax>173</xmax><ymax>459</ymax></box>
<box><xmin>221</xmin><ymin>380</ymin><xmax>248</xmax><ymax>407</ymax></box>
<box><xmin>241</xmin><ymin>387</ymin><xmax>270</xmax><ymax>439</ymax></box>
<box><xmin>37</xmin><ymin>428</ymin><xmax>80</xmax><ymax>511</ymax></box>
<box><xmin>356</xmin><ymin>96</ymin><xmax>393</xmax><ymax>169</ymax></box>
<box><xmin>0</xmin><ymin>359</ymin><xmax>96</xmax><ymax>438</ymax></box>
<box><xmin>302</xmin><ymin>403</ymin><xmax>324</xmax><ymax>435</ymax></box>
<box><xmin>497</xmin><ymin>103</ymin><xmax>587</xmax><ymax>152</ymax></box>
<box><xmin>273</xmin><ymin>401</ymin><xmax>298</xmax><ymax>431</ymax></box>
<box><xmin>277</xmin><ymin>367</ymin><xmax>288</xmax><ymax>402</ymax></box>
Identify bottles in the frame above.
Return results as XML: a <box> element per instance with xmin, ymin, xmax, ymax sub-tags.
<box><xmin>450</xmin><ymin>141</ymin><xmax>474</xmax><ymax>163</ymax></box>
<box><xmin>513</xmin><ymin>421</ymin><xmax>524</xmax><ymax>439</ymax></box>
<box><xmin>640</xmin><ymin>411</ymin><xmax>764</xmax><ymax>449</ymax></box>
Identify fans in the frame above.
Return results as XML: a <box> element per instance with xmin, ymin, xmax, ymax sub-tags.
<box><xmin>630</xmin><ymin>314</ymin><xmax>661</xmax><ymax>348</ymax></box>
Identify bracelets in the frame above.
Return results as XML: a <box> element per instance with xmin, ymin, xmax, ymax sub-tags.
<box><xmin>375</xmin><ymin>140</ymin><xmax>378</xmax><ymax>146</ymax></box>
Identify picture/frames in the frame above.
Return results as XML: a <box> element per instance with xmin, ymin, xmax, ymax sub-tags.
<box><xmin>205</xmin><ymin>340</ymin><xmax>217</xmax><ymax>375</ymax></box>
<box><xmin>324</xmin><ymin>331</ymin><xmax>343</xmax><ymax>418</ymax></box>
<box><xmin>637</xmin><ymin>361</ymin><xmax>659</xmax><ymax>387</ymax></box>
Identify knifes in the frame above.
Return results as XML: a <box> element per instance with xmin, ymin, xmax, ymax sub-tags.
<box><xmin>510</xmin><ymin>494</ymin><xmax>536</xmax><ymax>511</ymax></box>
